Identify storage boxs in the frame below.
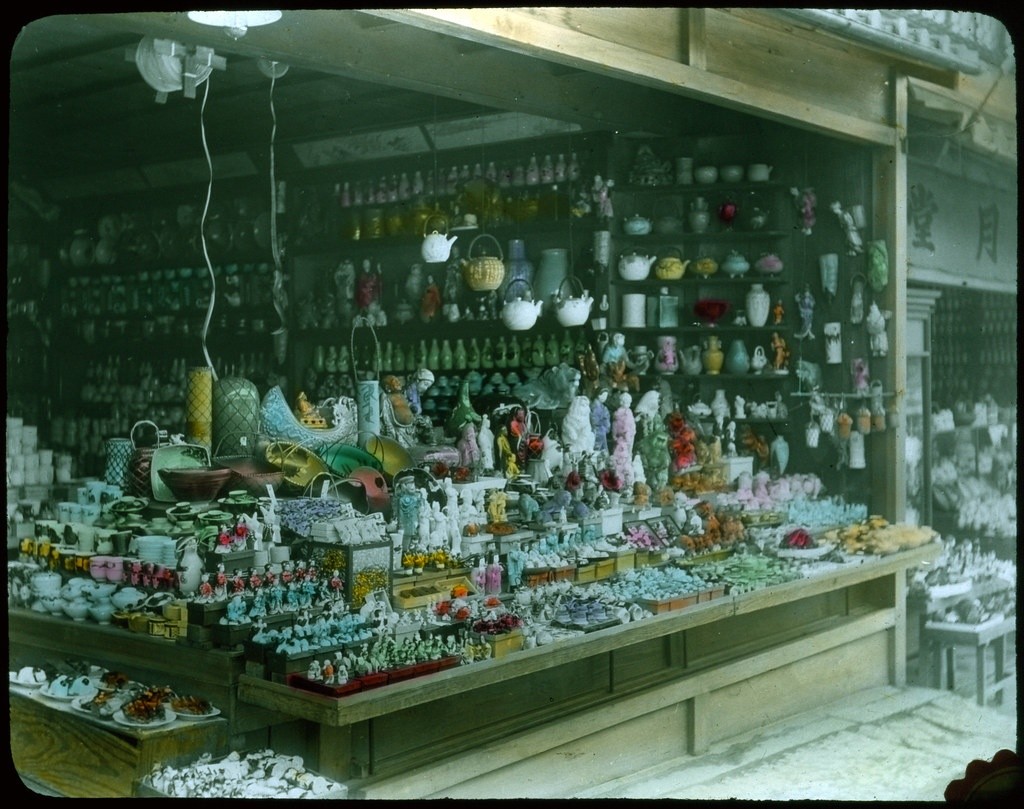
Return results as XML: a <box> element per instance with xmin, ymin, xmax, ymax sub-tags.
<box><xmin>189</xmin><ymin>489</ymin><xmax>786</xmax><ymax>698</ymax></box>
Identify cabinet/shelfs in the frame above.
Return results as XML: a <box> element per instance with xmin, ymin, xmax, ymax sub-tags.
<box><xmin>905</xmin><ymin>573</ymin><xmax>1016</xmax><ymax>706</ymax></box>
<box><xmin>54</xmin><ymin>177</ymin><xmax>790</xmax><ymax>459</ymax></box>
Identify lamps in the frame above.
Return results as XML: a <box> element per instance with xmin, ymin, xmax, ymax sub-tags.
<box><xmin>187</xmin><ymin>10</ymin><xmax>283</xmax><ymax>41</ymax></box>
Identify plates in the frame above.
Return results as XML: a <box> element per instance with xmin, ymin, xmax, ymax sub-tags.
<box><xmin>112</xmin><ymin>706</ymin><xmax>178</xmax><ymax>728</ymax></box>
<box><xmin>70</xmin><ymin>694</ymin><xmax>124</xmax><ymax>716</ymax></box>
<box><xmin>38</xmin><ymin>681</ymin><xmax>99</xmax><ymax>702</ymax></box>
<box><xmin>135</xmin><ymin>535</ymin><xmax>180</xmax><ymax>570</ymax></box>
<box><xmin>8</xmin><ymin>670</ymin><xmax>46</xmax><ymax>688</ymax></box>
<box><xmin>167</xmin><ymin>702</ymin><xmax>222</xmax><ymax>721</ymax></box>
<box><xmin>56</xmin><ymin>663</ymin><xmax>178</xmax><ymax>709</ymax></box>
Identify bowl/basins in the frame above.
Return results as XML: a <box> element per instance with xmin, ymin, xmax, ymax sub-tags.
<box><xmin>112</xmin><ymin>491</ymin><xmax>258</xmax><ymax>548</ymax></box>
<box><xmin>6</xmin><ymin>412</ymin><xmax>131</xmax><ymax>627</ymax></box>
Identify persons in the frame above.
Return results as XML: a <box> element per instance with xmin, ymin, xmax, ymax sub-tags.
<box><xmin>355</xmin><ymin>259</ymin><xmax>382</xmax><ymax>316</ymax></box>
<box><xmin>790</xmin><ymin>187</ymin><xmax>889</xmax><ymax>358</ymax></box>
<box><xmin>417</xmin><ymin>275</ymin><xmax>441</xmax><ymax>323</ymax></box>
<box><xmin>591</xmin><ymin>172</ymin><xmax>614</xmax><ymax>222</ymax></box>
<box><xmin>198</xmin><ymin>333</ymin><xmax>933</xmax><ymax>688</ymax></box>
